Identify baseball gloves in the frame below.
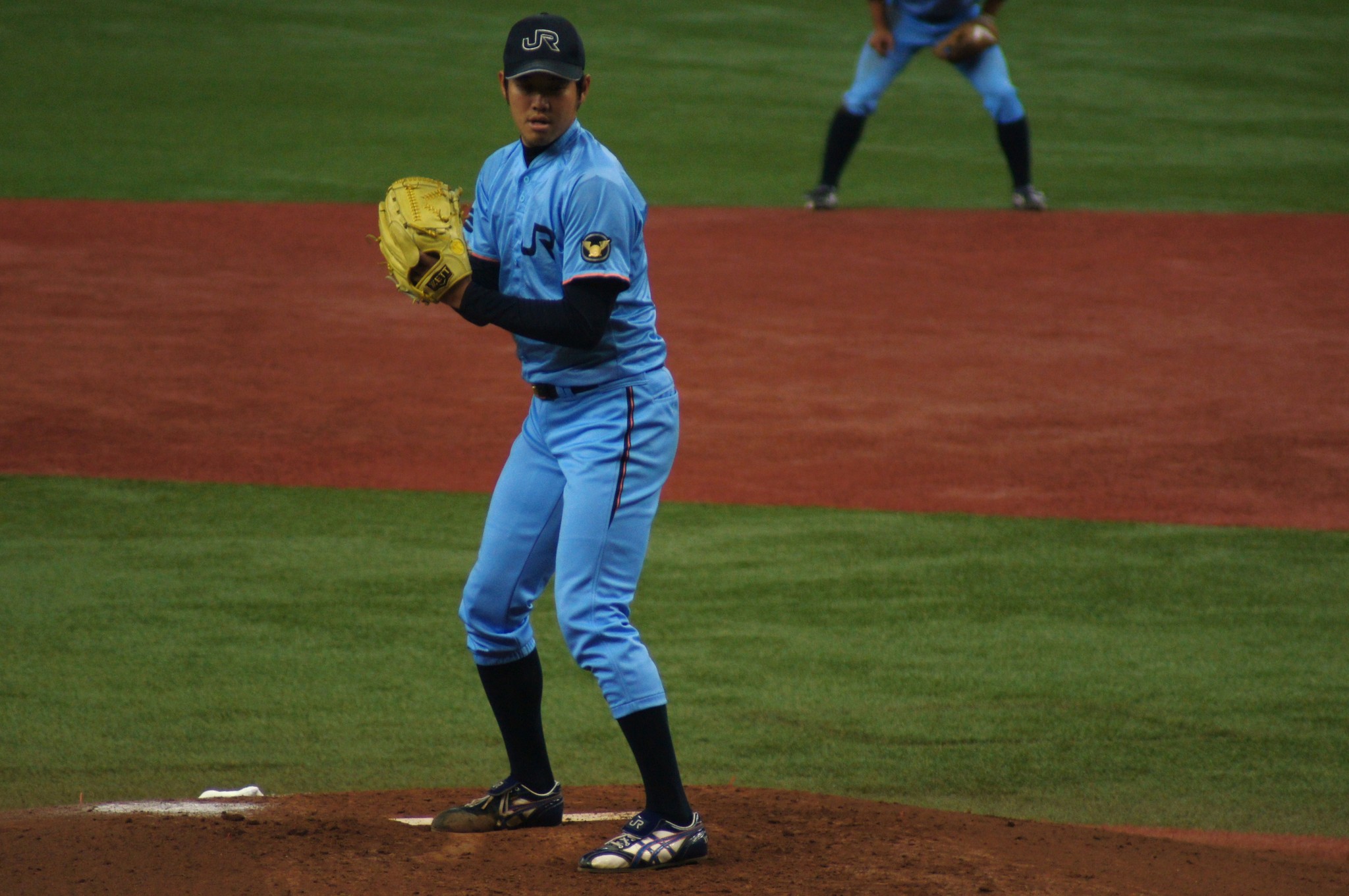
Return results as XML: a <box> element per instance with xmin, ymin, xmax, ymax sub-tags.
<box><xmin>934</xmin><ymin>14</ymin><xmax>998</xmax><ymax>64</ymax></box>
<box><xmin>377</xmin><ymin>176</ymin><xmax>475</xmax><ymax>305</ymax></box>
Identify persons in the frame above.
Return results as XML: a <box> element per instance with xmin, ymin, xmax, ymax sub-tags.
<box><xmin>399</xmin><ymin>11</ymin><xmax>721</xmax><ymax>873</ymax></box>
<box><xmin>801</xmin><ymin>0</ymin><xmax>1047</xmax><ymax>213</ymax></box>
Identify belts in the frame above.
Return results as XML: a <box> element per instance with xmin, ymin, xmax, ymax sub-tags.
<box><xmin>532</xmin><ymin>380</ymin><xmax>599</xmax><ymax>402</ymax></box>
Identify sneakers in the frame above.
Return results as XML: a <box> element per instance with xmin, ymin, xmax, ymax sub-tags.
<box><xmin>805</xmin><ymin>183</ymin><xmax>836</xmax><ymax>209</ymax></box>
<box><xmin>579</xmin><ymin>804</ymin><xmax>709</xmax><ymax>871</ymax></box>
<box><xmin>431</xmin><ymin>775</ymin><xmax>562</xmax><ymax>831</ymax></box>
<box><xmin>1012</xmin><ymin>184</ymin><xmax>1046</xmax><ymax>210</ymax></box>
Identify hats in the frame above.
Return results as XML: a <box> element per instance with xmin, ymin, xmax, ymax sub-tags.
<box><xmin>502</xmin><ymin>10</ymin><xmax>585</xmax><ymax>81</ymax></box>
